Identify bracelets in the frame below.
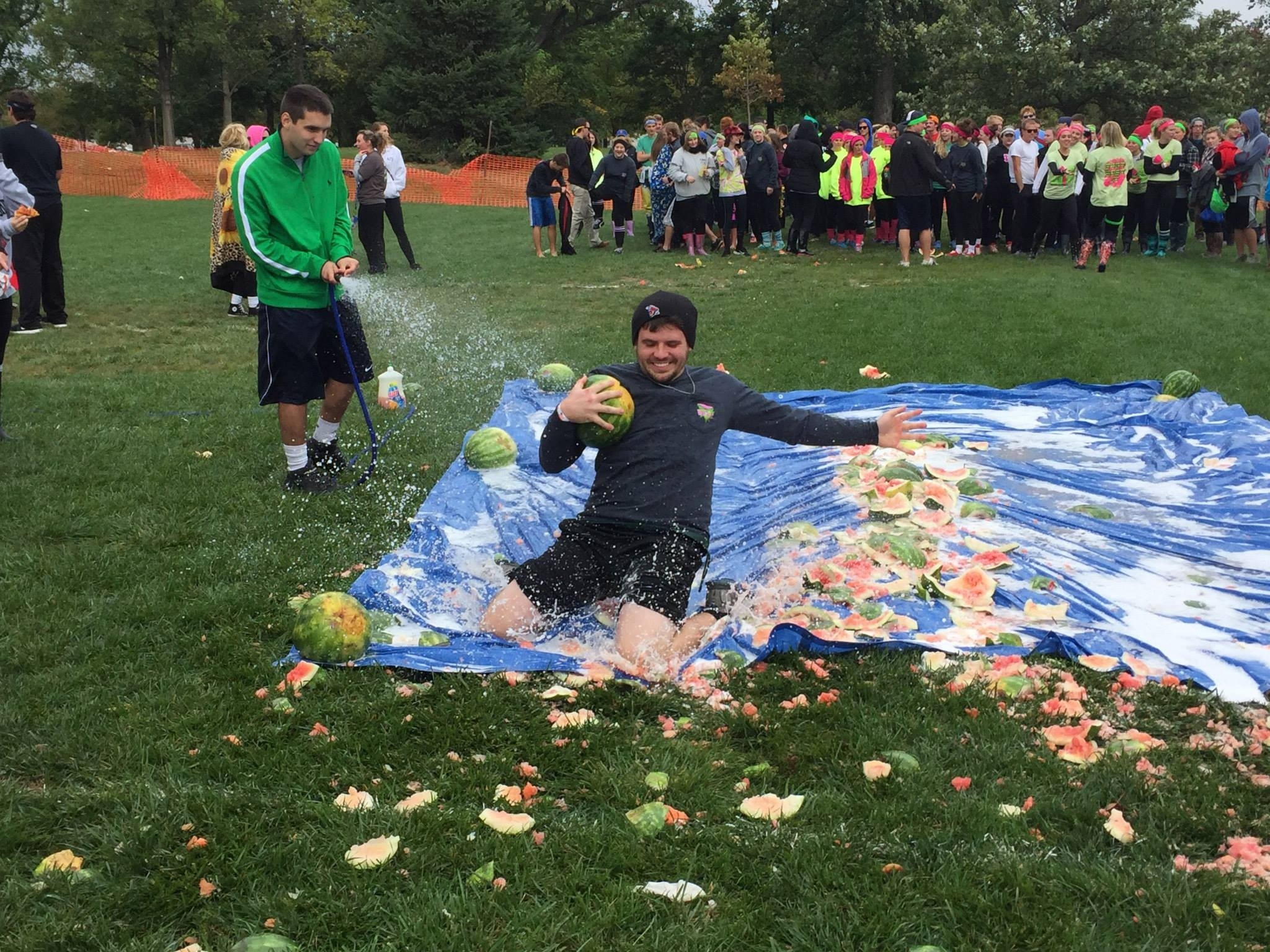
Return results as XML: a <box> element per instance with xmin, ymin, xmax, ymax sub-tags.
<box><xmin>557</xmin><ymin>403</ymin><xmax>571</xmax><ymax>422</ymax></box>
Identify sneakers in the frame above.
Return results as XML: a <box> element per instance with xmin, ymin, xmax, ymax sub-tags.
<box><xmin>285</xmin><ymin>458</ymin><xmax>345</xmax><ymax>493</ymax></box>
<box><xmin>40</xmin><ymin>316</ymin><xmax>67</xmax><ymax>327</ymax></box>
<box><xmin>704</xmin><ymin>577</ymin><xmax>741</xmax><ymax>614</ymax></box>
<box><xmin>306</xmin><ymin>438</ymin><xmax>350</xmax><ymax>471</ymax></box>
<box><xmin>9</xmin><ymin>324</ymin><xmax>42</xmax><ymax>334</ymax></box>
<box><xmin>495</xmin><ymin>558</ymin><xmax>520</xmax><ymax>577</ymax></box>
<box><xmin>228</xmin><ymin>303</ymin><xmax>248</xmax><ymax>316</ymax></box>
<box><xmin>248</xmin><ymin>303</ymin><xmax>260</xmax><ymax>315</ymax></box>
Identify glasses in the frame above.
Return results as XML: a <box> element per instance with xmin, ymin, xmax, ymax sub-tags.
<box><xmin>1023</xmin><ymin>128</ymin><xmax>1037</xmax><ymax>133</ymax></box>
<box><xmin>584</xmin><ymin>127</ymin><xmax>590</xmax><ymax>130</ymax></box>
<box><xmin>1023</xmin><ymin>114</ymin><xmax>1035</xmax><ymax>118</ymax></box>
<box><xmin>1192</xmin><ymin>125</ymin><xmax>1203</xmax><ymax>128</ymax></box>
<box><xmin>859</xmin><ymin>126</ymin><xmax>868</xmax><ymax>129</ymax></box>
<box><xmin>263</xmin><ymin>136</ymin><xmax>266</xmax><ymax>140</ymax></box>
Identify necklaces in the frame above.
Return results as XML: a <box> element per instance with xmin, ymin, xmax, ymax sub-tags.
<box><xmin>650</xmin><ymin>364</ymin><xmax>696</xmax><ymax>395</ymax></box>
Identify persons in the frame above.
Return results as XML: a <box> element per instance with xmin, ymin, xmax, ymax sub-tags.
<box><xmin>946</xmin><ymin>104</ymin><xmax>1270</xmax><ymax>274</ymax></box>
<box><xmin>232</xmin><ymin>86</ymin><xmax>373</xmax><ymax>494</ymax></box>
<box><xmin>208</xmin><ymin>122</ymin><xmax>270</xmax><ymax>318</ymax></box>
<box><xmin>778</xmin><ymin>110</ymin><xmax>958</xmax><ymax>266</ymax></box>
<box><xmin>0</xmin><ymin>153</ymin><xmax>34</xmax><ymax>393</ymax></box>
<box><xmin>0</xmin><ymin>90</ymin><xmax>68</xmax><ymax>334</ymax></box>
<box><xmin>560</xmin><ymin>114</ymin><xmax>785</xmax><ymax>259</ymax></box>
<box><xmin>343</xmin><ymin>121</ymin><xmax>422</xmax><ymax>274</ymax></box>
<box><xmin>475</xmin><ymin>289</ymin><xmax>930</xmax><ymax>667</ymax></box>
<box><xmin>526</xmin><ymin>153</ymin><xmax>571</xmax><ymax>258</ymax></box>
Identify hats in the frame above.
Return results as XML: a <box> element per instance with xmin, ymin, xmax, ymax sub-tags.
<box><xmin>906</xmin><ymin>110</ymin><xmax>927</xmax><ymax>126</ymax></box>
<box><xmin>631</xmin><ymin>290</ymin><xmax>698</xmax><ymax>349</ymax></box>
<box><xmin>928</xmin><ymin>115</ymin><xmax>939</xmax><ymax>124</ymax></box>
<box><xmin>617</xmin><ymin>129</ymin><xmax>628</xmax><ymax>137</ymax></box>
<box><xmin>724</xmin><ymin>126</ymin><xmax>742</xmax><ymax>135</ymax></box>
<box><xmin>1002</xmin><ymin>125</ymin><xmax>1014</xmax><ymax>136</ymax></box>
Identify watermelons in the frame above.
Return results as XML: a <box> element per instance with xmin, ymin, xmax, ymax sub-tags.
<box><xmin>293</xmin><ymin>591</ymin><xmax>373</xmax><ymax>665</ymax></box>
<box><xmin>1161</xmin><ymin>370</ymin><xmax>1200</xmax><ymax>398</ymax></box>
<box><xmin>577</xmin><ymin>374</ymin><xmax>635</xmax><ymax>449</ymax></box>
<box><xmin>463</xmin><ymin>427</ymin><xmax>517</xmax><ymax>469</ymax></box>
<box><xmin>535</xmin><ymin>363</ymin><xmax>575</xmax><ymax>393</ymax></box>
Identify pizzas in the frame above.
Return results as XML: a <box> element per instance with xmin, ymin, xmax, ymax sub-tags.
<box><xmin>14</xmin><ymin>205</ymin><xmax>40</xmax><ymax>218</ymax></box>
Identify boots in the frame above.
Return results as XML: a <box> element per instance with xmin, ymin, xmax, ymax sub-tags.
<box><xmin>1074</xmin><ymin>238</ymin><xmax>1094</xmax><ymax>268</ymax></box>
<box><xmin>1097</xmin><ymin>240</ymin><xmax>1113</xmax><ymax>273</ymax></box>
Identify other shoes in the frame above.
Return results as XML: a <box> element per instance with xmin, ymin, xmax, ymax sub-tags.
<box><xmin>588</xmin><ymin>220</ymin><xmax>1266</xmax><ymax>266</ymax></box>
<box><xmin>410</xmin><ymin>262</ymin><xmax>421</xmax><ymax>272</ymax></box>
<box><xmin>536</xmin><ymin>252</ymin><xmax>544</xmax><ymax>258</ymax></box>
<box><xmin>551</xmin><ymin>250</ymin><xmax>558</xmax><ymax>257</ymax></box>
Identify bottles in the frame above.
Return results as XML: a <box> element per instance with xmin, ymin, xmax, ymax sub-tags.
<box><xmin>377</xmin><ymin>367</ymin><xmax>406</xmax><ymax>409</ymax></box>
